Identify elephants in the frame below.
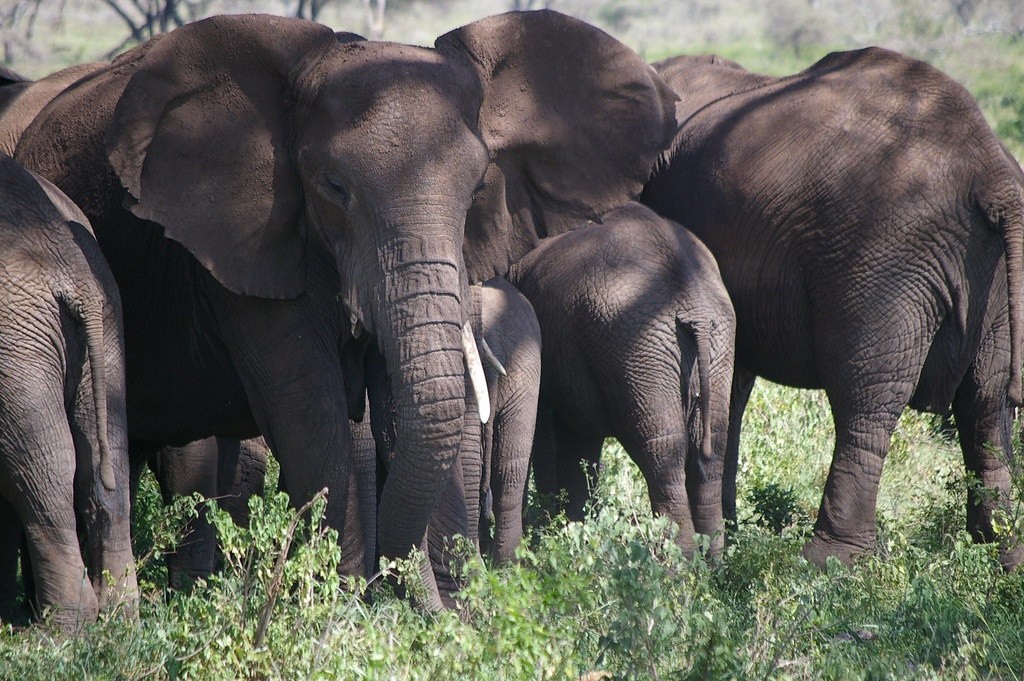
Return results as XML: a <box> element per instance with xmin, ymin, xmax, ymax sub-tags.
<box><xmin>641</xmin><ymin>43</ymin><xmax>1024</xmax><ymax>564</ymax></box>
<box><xmin>1</xmin><ymin>8</ymin><xmax>679</xmax><ymax>634</ymax></box>
<box><xmin>505</xmin><ymin>198</ymin><xmax>737</xmax><ymax>567</ymax></box>
